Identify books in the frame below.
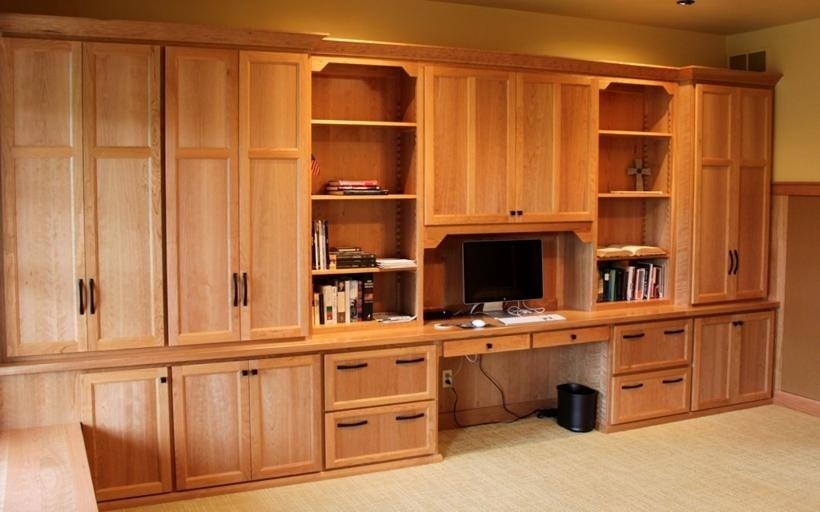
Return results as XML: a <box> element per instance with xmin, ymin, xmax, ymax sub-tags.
<box><xmin>312</xmin><ymin>218</ymin><xmax>377</xmax><ymax>270</ymax></box>
<box><xmin>327</xmin><ymin>179</ymin><xmax>390</xmax><ymax>195</ymax></box>
<box><xmin>313</xmin><ymin>274</ymin><xmax>375</xmax><ymax>327</ymax></box>
<box><xmin>374</xmin><ymin>310</ymin><xmax>416</xmax><ymax>325</ymax></box>
<box><xmin>598</xmin><ymin>262</ymin><xmax>664</xmax><ymax>302</ymax></box>
<box><xmin>376</xmin><ymin>258</ymin><xmax>418</xmax><ymax>270</ymax></box>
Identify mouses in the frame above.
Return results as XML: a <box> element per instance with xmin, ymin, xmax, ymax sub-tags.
<box><xmin>473</xmin><ymin>319</ymin><xmax>487</xmax><ymax>327</ymax></box>
<box><xmin>458</xmin><ymin>322</ymin><xmax>474</xmax><ymax>328</ymax></box>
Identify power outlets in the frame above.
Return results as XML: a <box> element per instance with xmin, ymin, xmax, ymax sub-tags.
<box><xmin>442</xmin><ymin>370</ymin><xmax>452</xmax><ymax>388</ymax></box>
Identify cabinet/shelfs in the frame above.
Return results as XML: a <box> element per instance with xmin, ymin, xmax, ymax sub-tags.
<box><xmin>310</xmin><ymin>42</ymin><xmax>786</xmax><ymax>477</ymax></box>
<box><xmin>162</xmin><ymin>9</ymin><xmax>327</xmax><ymax>482</ymax></box>
<box><xmin>1</xmin><ymin>13</ymin><xmax>176</xmax><ymax>512</ymax></box>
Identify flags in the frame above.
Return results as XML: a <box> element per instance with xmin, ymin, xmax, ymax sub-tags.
<box><xmin>311</xmin><ymin>154</ymin><xmax>322</xmax><ymax>176</ymax></box>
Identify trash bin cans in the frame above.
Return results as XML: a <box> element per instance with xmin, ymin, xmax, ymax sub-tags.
<box><xmin>557</xmin><ymin>383</ymin><xmax>599</xmax><ymax>432</ymax></box>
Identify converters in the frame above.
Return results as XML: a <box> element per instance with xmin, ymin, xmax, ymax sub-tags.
<box><xmin>424</xmin><ymin>310</ymin><xmax>454</xmax><ymax>320</ymax></box>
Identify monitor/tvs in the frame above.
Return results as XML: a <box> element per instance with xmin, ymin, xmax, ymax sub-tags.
<box><xmin>462</xmin><ymin>238</ymin><xmax>544</xmax><ymax>317</ymax></box>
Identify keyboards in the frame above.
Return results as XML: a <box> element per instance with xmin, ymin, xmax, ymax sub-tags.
<box><xmin>497</xmin><ymin>313</ymin><xmax>566</xmax><ymax>324</ymax></box>
